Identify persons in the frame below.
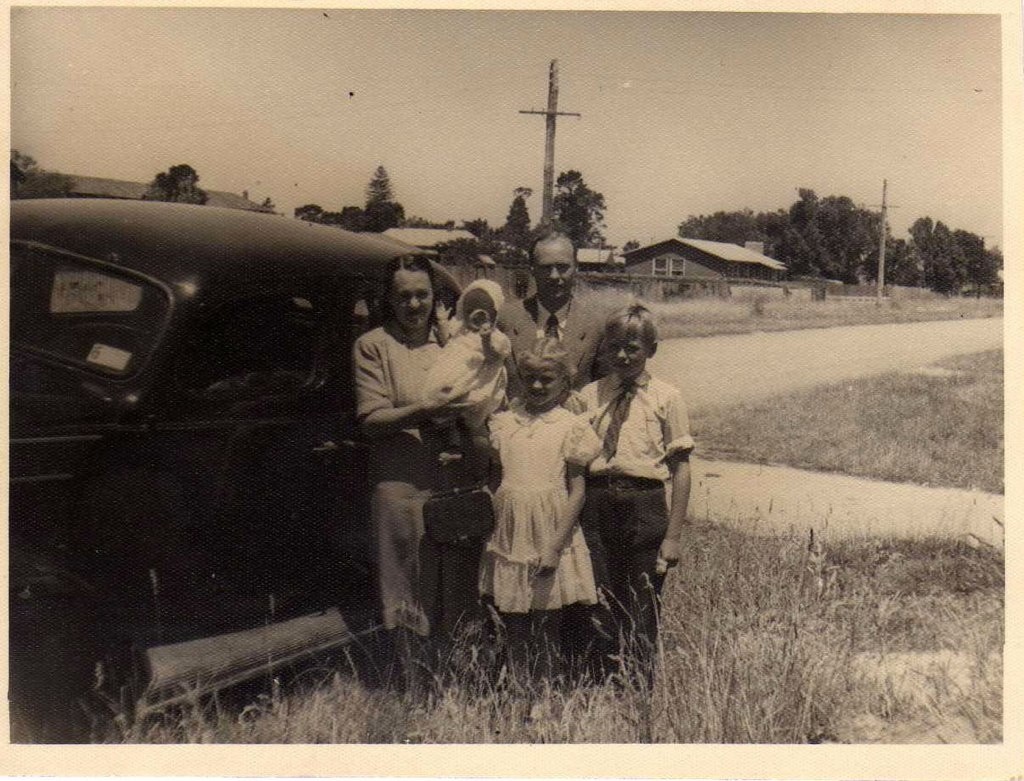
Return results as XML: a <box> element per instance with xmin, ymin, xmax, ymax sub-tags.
<box><xmin>498</xmin><ymin>232</ymin><xmax>609</xmax><ymax>407</ymax></box>
<box><xmin>476</xmin><ymin>337</ymin><xmax>603</xmax><ymax>689</ymax></box>
<box><xmin>353</xmin><ymin>251</ymin><xmax>477</xmax><ymax>714</ymax></box>
<box><xmin>580</xmin><ymin>302</ymin><xmax>695</xmax><ymax>699</ymax></box>
<box><xmin>419</xmin><ymin>278</ymin><xmax>512</xmax><ymax>462</ymax></box>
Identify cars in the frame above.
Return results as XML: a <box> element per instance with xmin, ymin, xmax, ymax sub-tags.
<box><xmin>8</xmin><ymin>202</ymin><xmax>464</xmax><ymax>723</ymax></box>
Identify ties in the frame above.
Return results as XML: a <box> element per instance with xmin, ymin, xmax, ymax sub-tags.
<box><xmin>602</xmin><ymin>379</ymin><xmax>637</xmax><ymax>463</ymax></box>
<box><xmin>544</xmin><ymin>315</ymin><xmax>560</xmax><ymax>342</ymax></box>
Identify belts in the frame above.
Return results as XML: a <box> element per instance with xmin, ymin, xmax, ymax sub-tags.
<box><xmin>589</xmin><ymin>473</ymin><xmax>664</xmax><ymax>491</ymax></box>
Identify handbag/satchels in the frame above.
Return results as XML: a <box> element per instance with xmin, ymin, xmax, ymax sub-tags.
<box><xmin>422</xmin><ymin>485</ymin><xmax>495</xmax><ymax>544</ymax></box>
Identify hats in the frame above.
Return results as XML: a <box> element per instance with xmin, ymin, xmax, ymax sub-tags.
<box><xmin>455</xmin><ymin>278</ymin><xmax>505</xmax><ymax>326</ymax></box>
